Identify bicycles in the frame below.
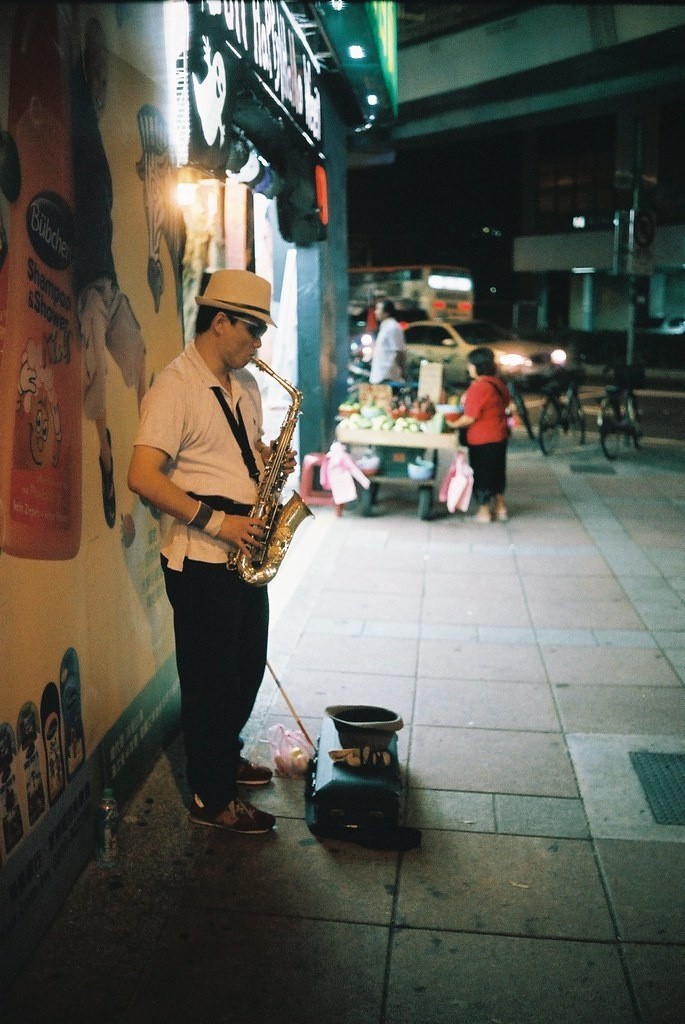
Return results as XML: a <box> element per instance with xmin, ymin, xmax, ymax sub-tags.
<box><xmin>500</xmin><ymin>372</ymin><xmax>535</xmax><ymax>442</ymax></box>
<box><xmin>594</xmin><ymin>360</ymin><xmax>646</xmax><ymax>460</ymax></box>
<box><xmin>536</xmin><ymin>354</ymin><xmax>588</xmax><ymax>456</ymax></box>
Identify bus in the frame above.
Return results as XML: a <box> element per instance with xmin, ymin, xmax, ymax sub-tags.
<box><xmin>348</xmin><ymin>264</ymin><xmax>475</xmax><ymax>324</ymax></box>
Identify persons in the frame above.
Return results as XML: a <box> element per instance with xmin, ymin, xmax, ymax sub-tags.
<box><xmin>128</xmin><ymin>268</ymin><xmax>295</xmax><ymax>833</ymax></box>
<box><xmin>445</xmin><ymin>347</ymin><xmax>509</xmax><ymax>523</ymax></box>
<box><xmin>70</xmin><ymin>1</ymin><xmax>155</xmax><ymax>527</ymax></box>
<box><xmin>367</xmin><ymin>300</ymin><xmax>409</xmax><ymax>396</ymax></box>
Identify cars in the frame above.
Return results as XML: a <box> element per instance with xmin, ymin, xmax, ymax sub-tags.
<box><xmin>351</xmin><ymin>297</ymin><xmax>426</xmax><ymax>325</ymax></box>
<box><xmin>401</xmin><ymin>317</ymin><xmax>569</xmax><ymax>395</ymax></box>
<box><xmin>638</xmin><ymin>314</ymin><xmax>685</xmax><ymax>336</ymax></box>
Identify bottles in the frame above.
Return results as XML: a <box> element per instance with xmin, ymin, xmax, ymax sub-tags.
<box><xmin>94</xmin><ymin>788</ymin><xmax>118</xmax><ymax>863</ymax></box>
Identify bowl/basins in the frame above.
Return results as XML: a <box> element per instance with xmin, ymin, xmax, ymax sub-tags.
<box><xmin>407</xmin><ymin>461</ymin><xmax>432</xmax><ymax>478</ymax></box>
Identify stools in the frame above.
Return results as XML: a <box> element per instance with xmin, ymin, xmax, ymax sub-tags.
<box><xmin>299</xmin><ymin>452</ymin><xmax>346</xmax><ymax>518</ymax></box>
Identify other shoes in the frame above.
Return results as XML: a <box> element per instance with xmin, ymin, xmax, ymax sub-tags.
<box><xmin>463</xmin><ymin>509</ymin><xmax>507</xmax><ymax>524</ymax></box>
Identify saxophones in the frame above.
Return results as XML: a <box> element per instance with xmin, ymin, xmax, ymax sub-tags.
<box><xmin>228</xmin><ymin>355</ymin><xmax>317</xmax><ymax>586</ymax></box>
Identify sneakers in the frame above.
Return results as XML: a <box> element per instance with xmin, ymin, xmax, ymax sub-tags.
<box><xmin>235</xmin><ymin>757</ymin><xmax>273</xmax><ymax>785</ymax></box>
<box><xmin>187</xmin><ymin>797</ymin><xmax>277</xmax><ymax>834</ymax></box>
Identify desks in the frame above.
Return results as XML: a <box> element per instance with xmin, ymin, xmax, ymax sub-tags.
<box><xmin>336</xmin><ymin>417</ymin><xmax>459</xmax><ymax>521</ymax></box>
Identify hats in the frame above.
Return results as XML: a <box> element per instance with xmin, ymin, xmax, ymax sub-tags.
<box><xmin>194</xmin><ymin>268</ymin><xmax>278</xmax><ymax>329</ymax></box>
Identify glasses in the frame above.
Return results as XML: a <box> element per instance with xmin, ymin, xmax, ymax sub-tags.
<box><xmin>233</xmin><ymin>316</ymin><xmax>267</xmax><ymax>339</ymax></box>
